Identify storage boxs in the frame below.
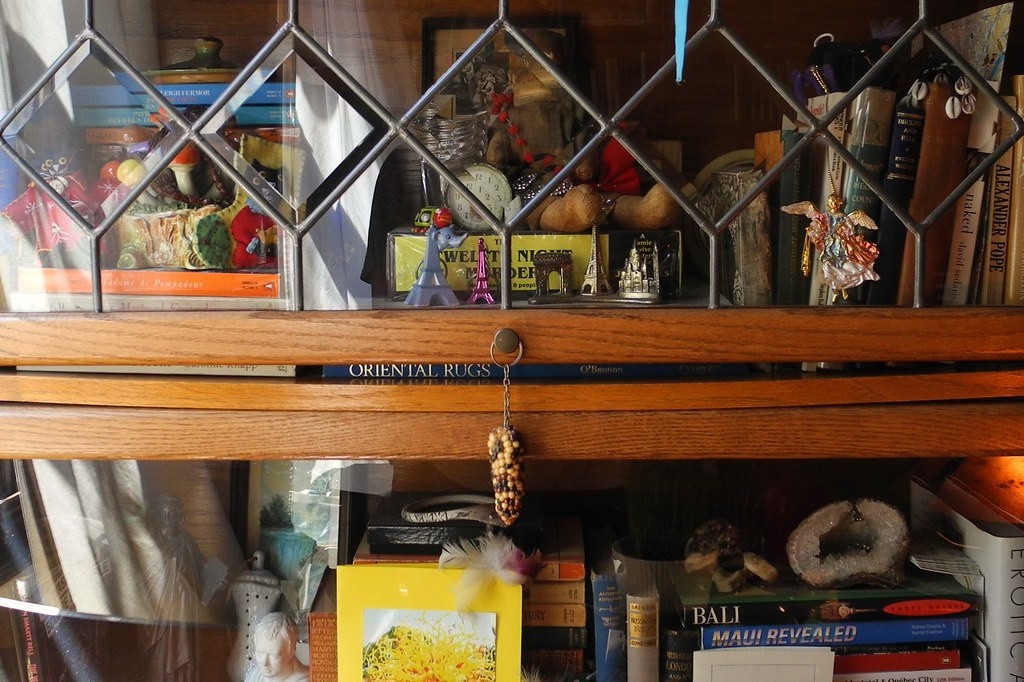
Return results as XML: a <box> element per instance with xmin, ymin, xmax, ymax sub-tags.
<box><xmin>14</xmin><ymin>264</ymin><xmax>297</xmax><ymax>378</ymax></box>
<box><xmin>911</xmin><ymin>457</ymin><xmax>1024</xmax><ymax>682</ymax></box>
<box><xmin>386</xmin><ymin>223</ymin><xmax>610</xmax><ymax>299</ymax></box>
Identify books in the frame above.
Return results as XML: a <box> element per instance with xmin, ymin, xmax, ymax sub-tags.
<box><xmin>334</xmin><ymin>471</ymin><xmax>1024</xmax><ymax>682</ymax></box>
<box><xmin>15</xmin><ymin>554</ymin><xmax>96</xmax><ymax>682</ymax></box>
<box><xmin>690</xmin><ymin>54</ymin><xmax>1024</xmax><ymax>374</ymax></box>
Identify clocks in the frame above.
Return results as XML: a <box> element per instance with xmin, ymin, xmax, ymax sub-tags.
<box><xmin>442</xmin><ymin>163</ymin><xmax>511</xmax><ymax>232</ymax></box>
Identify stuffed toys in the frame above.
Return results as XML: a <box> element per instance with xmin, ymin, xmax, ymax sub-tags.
<box><xmin>486</xmin><ymin>28</ymin><xmax>682</xmax><ymax>233</ymax></box>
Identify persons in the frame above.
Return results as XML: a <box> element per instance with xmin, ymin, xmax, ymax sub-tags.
<box><xmin>136</xmin><ymin>495</ymin><xmax>228</xmax><ymax>682</ymax></box>
<box><xmin>244</xmin><ymin>612</ymin><xmax>310</xmax><ymax>681</ymax></box>
<box><xmin>810</xmin><ymin>194</ymin><xmax>880</xmax><ymax>301</ymax></box>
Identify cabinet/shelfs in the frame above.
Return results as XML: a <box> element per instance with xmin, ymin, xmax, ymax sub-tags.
<box><xmin>0</xmin><ymin>0</ymin><xmax>1024</xmax><ymax>682</ymax></box>
